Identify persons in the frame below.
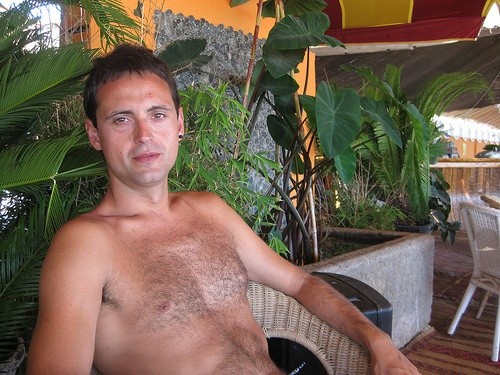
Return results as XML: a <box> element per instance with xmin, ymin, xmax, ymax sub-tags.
<box><xmin>24</xmin><ymin>43</ymin><xmax>423</xmax><ymax>375</ymax></box>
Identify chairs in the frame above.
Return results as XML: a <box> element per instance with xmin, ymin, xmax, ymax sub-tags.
<box><xmin>90</xmin><ymin>272</ymin><xmax>393</xmax><ymax>375</ymax></box>
<box><xmin>447</xmin><ymin>202</ymin><xmax>500</xmax><ymax>361</ymax></box>
